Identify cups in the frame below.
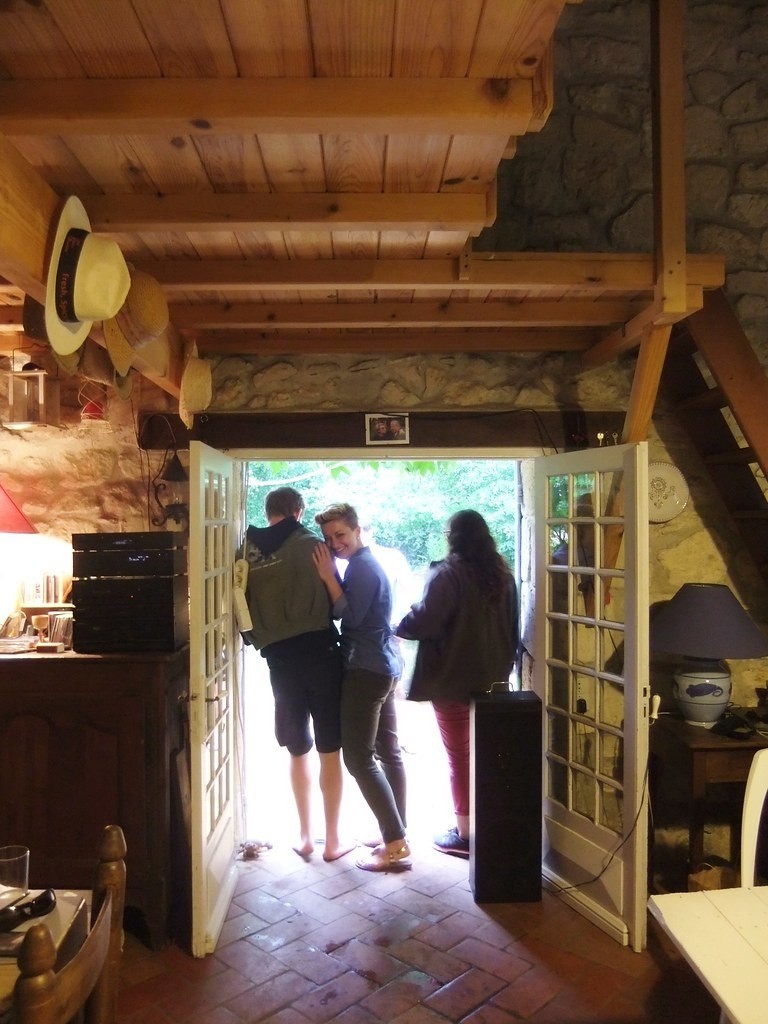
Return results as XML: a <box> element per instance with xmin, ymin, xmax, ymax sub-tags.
<box><xmin>0</xmin><ymin>845</ymin><xmax>30</xmax><ymax>906</ymax></box>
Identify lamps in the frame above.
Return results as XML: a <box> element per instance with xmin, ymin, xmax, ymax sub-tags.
<box><xmin>646</xmin><ymin>583</ymin><xmax>768</xmax><ymax>728</ymax></box>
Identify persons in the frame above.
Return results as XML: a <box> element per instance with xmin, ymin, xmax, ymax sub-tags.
<box><xmin>375</xmin><ymin>419</ymin><xmax>406</xmax><ymax>440</ymax></box>
<box><xmin>392</xmin><ymin>508</ymin><xmax>521</xmax><ymax>859</ymax></box>
<box><xmin>234</xmin><ymin>487</ymin><xmax>356</xmax><ymax>861</ymax></box>
<box><xmin>553</xmin><ymin>492</ymin><xmax>601</xmax><ymax>663</ymax></box>
<box><xmin>312</xmin><ymin>502</ymin><xmax>412</xmax><ymax>870</ymax></box>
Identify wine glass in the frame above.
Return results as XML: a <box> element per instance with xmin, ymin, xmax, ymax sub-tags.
<box><xmin>31</xmin><ymin>615</ymin><xmax>48</xmax><ymax>642</ymax></box>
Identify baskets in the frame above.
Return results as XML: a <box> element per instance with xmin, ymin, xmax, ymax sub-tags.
<box><xmin>687</xmin><ymin>866</ymin><xmax>741</xmax><ymax>891</ymax></box>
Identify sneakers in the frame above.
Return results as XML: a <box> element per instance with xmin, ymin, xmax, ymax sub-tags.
<box><xmin>432</xmin><ymin>826</ymin><xmax>469</xmax><ymax>855</ymax></box>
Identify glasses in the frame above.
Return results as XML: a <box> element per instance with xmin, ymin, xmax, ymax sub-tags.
<box><xmin>0</xmin><ymin>888</ymin><xmax>56</xmax><ymax>933</ymax></box>
<box><xmin>380</xmin><ymin>426</ymin><xmax>387</xmax><ymax>429</ymax></box>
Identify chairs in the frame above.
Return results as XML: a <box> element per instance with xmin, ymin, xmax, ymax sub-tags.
<box><xmin>16</xmin><ymin>825</ymin><xmax>128</xmax><ymax>1024</ymax></box>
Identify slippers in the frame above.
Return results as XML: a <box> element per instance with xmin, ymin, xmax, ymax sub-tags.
<box><xmin>356</xmin><ymin>850</ymin><xmax>412</xmax><ymax>870</ymax></box>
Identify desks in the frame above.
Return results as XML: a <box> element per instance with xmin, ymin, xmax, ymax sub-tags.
<box><xmin>650</xmin><ymin>708</ymin><xmax>768</xmax><ymax>870</ymax></box>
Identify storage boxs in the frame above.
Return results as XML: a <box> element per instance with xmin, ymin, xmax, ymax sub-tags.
<box><xmin>647</xmin><ymin>866</ymin><xmax>742</xmax><ymax>970</ymax></box>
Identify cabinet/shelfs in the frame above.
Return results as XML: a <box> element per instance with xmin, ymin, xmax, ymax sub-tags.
<box><xmin>0</xmin><ymin>644</ymin><xmax>191</xmax><ymax>949</ymax></box>
